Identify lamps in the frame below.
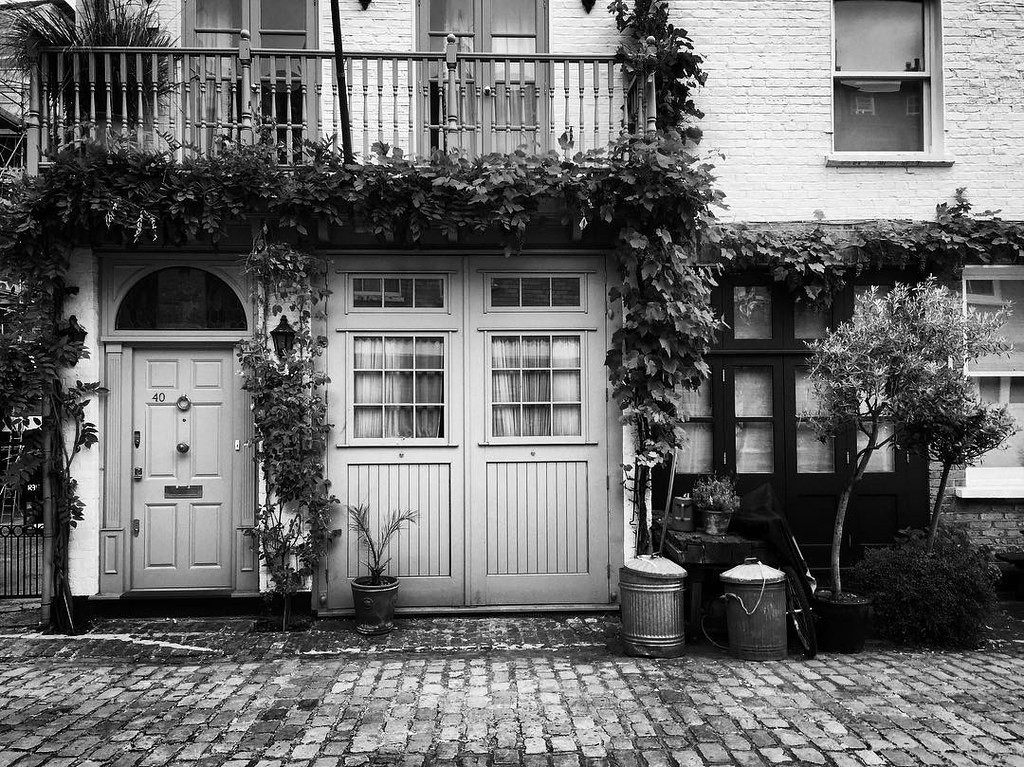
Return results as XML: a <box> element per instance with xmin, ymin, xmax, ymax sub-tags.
<box><xmin>268</xmin><ymin>314</ymin><xmax>298</xmax><ymax>360</ymax></box>
<box><xmin>57</xmin><ymin>315</ymin><xmax>88</xmax><ymax>366</ymax></box>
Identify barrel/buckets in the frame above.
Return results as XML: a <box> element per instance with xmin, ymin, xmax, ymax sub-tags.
<box><xmin>618</xmin><ymin>551</ymin><xmax>687</xmax><ymax>659</ymax></box>
<box><xmin>720</xmin><ymin>557</ymin><xmax>789</xmax><ymax>661</ymax></box>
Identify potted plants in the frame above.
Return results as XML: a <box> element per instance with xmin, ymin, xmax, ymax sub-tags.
<box><xmin>789</xmin><ymin>270</ymin><xmax>1011</xmax><ymax>654</ymax></box>
<box><xmin>342</xmin><ymin>504</ymin><xmax>421</xmax><ymax>637</ymax></box>
<box><xmin>692</xmin><ymin>467</ymin><xmax>743</xmax><ymax>536</ymax></box>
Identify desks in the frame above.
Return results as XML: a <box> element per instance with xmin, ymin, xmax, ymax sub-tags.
<box><xmin>653</xmin><ymin>509</ymin><xmax>752</xmax><ymax>648</ymax></box>
